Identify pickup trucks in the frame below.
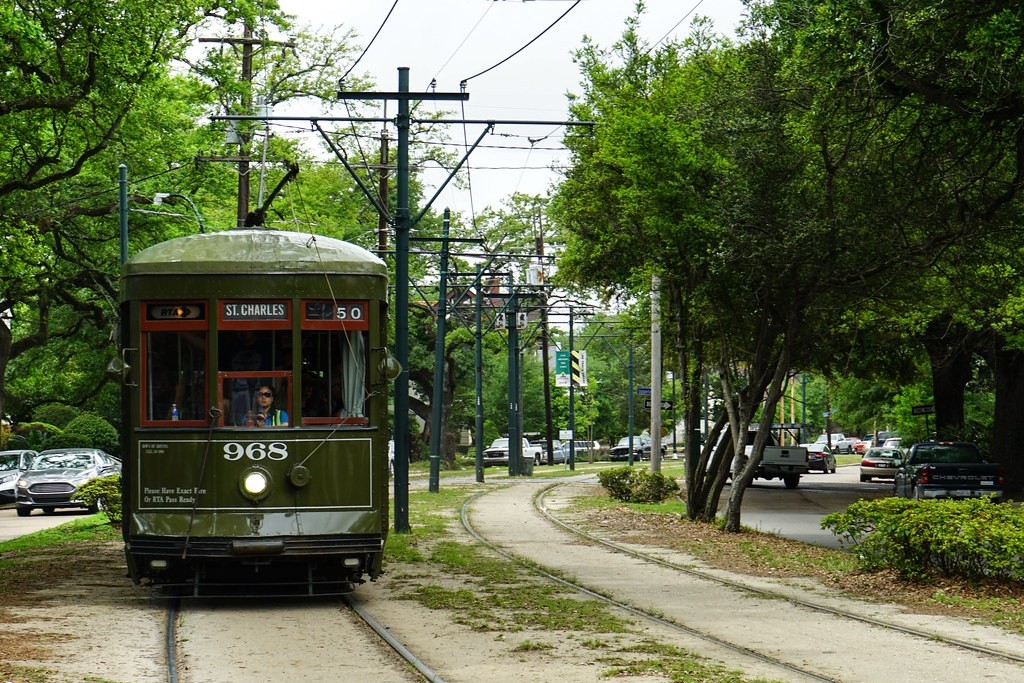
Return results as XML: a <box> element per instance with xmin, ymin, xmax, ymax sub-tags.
<box><xmin>707</xmin><ymin>430</ymin><xmax>811</xmax><ymax>489</ymax></box>
<box><xmin>609</xmin><ymin>437</ymin><xmax>666</xmax><ymax>463</ymax></box>
<box><xmin>815</xmin><ymin>433</ymin><xmax>853</xmax><ymax>454</ymax></box>
<box><xmin>483</xmin><ymin>438</ymin><xmax>543</xmax><ymax>467</ymax></box>
<box><xmin>891</xmin><ymin>442</ymin><xmax>1005</xmax><ymax>503</ymax></box>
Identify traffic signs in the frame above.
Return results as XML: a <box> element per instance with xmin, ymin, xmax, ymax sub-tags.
<box><xmin>644</xmin><ymin>399</ymin><xmax>673</xmax><ymax>410</ymax></box>
<box><xmin>912</xmin><ymin>405</ymin><xmax>936</xmax><ymax>417</ymax></box>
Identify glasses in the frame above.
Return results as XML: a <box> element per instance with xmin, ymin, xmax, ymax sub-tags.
<box><xmin>256</xmin><ymin>392</ymin><xmax>273</xmax><ymax>398</ymax></box>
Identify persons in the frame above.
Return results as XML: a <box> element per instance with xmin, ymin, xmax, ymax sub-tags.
<box><xmin>301</xmin><ymin>371</ymin><xmax>339</xmax><ymax>417</ymax></box>
<box><xmin>241</xmin><ymin>383</ymin><xmax>289</xmax><ymax>427</ymax></box>
<box><xmin>168</xmin><ymin>360</ymin><xmax>232</xmax><ymax>427</ymax></box>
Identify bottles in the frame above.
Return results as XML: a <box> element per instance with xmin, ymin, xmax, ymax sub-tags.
<box><xmin>171</xmin><ymin>403</ymin><xmax>179</xmax><ymax>420</ymax></box>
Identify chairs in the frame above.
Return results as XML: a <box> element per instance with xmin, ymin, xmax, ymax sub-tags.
<box><xmin>892</xmin><ymin>453</ymin><xmax>899</xmax><ymax>458</ymax></box>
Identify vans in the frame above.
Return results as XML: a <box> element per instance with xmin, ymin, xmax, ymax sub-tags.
<box><xmin>562</xmin><ymin>441</ymin><xmax>601</xmax><ymax>451</ymax></box>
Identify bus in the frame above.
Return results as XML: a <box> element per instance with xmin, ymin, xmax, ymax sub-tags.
<box><xmin>115</xmin><ymin>229</ymin><xmax>390</xmax><ymax>599</ymax></box>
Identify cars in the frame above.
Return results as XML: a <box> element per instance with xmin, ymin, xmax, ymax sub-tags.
<box><xmin>14</xmin><ymin>448</ymin><xmax>119</xmax><ymax>517</ymax></box>
<box><xmin>883</xmin><ymin>438</ymin><xmax>912</xmax><ymax>456</ymax></box>
<box><xmin>528</xmin><ymin>440</ymin><xmax>574</xmax><ymax>467</ymax></box>
<box><xmin>0</xmin><ymin>450</ymin><xmax>38</xmax><ymax>502</ymax></box>
<box><xmin>796</xmin><ymin>442</ymin><xmax>838</xmax><ymax>474</ymax></box>
<box><xmin>845</xmin><ymin>437</ymin><xmax>864</xmax><ymax>454</ymax></box>
<box><xmin>862</xmin><ymin>431</ymin><xmax>894</xmax><ymax>452</ymax></box>
<box><xmin>859</xmin><ymin>447</ymin><xmax>905</xmax><ymax>482</ymax></box>
